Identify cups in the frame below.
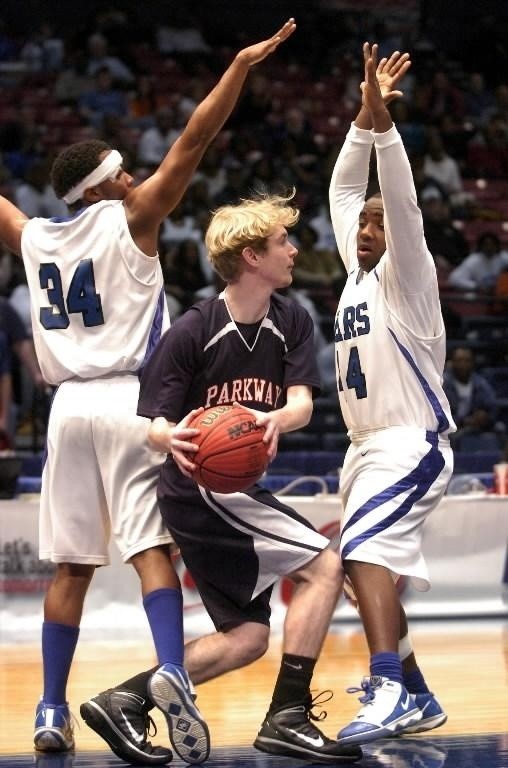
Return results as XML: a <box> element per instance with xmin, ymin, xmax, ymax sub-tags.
<box><xmin>493</xmin><ymin>462</ymin><xmax>508</xmax><ymax>495</ymax></box>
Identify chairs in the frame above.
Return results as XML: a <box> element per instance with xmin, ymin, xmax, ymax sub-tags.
<box><xmin>0</xmin><ymin>284</ymin><xmax>508</xmax><ymax>500</ymax></box>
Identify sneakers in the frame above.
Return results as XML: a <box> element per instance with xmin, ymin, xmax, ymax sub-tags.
<box><xmin>33</xmin><ymin>700</ymin><xmax>79</xmax><ymax>753</ymax></box>
<box><xmin>80</xmin><ymin>687</ymin><xmax>173</xmax><ymax>767</ymax></box>
<box><xmin>336</xmin><ymin>675</ymin><xmax>448</xmax><ymax>747</ymax></box>
<box><xmin>253</xmin><ymin>691</ymin><xmax>363</xmax><ymax>762</ymax></box>
<box><xmin>146</xmin><ymin>663</ymin><xmax>211</xmax><ymax>764</ymax></box>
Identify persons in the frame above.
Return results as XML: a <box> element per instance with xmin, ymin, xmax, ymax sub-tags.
<box><xmin>80</xmin><ymin>186</ymin><xmax>363</xmax><ymax>767</ymax></box>
<box><xmin>1</xmin><ymin>18</ymin><xmax>298</xmax><ymax>765</ymax></box>
<box><xmin>3</xmin><ymin>2</ymin><xmax>508</xmax><ymax>455</ymax></box>
<box><xmin>328</xmin><ymin>42</ymin><xmax>457</xmax><ymax>744</ymax></box>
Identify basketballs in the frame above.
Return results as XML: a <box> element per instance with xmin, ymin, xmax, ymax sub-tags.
<box><xmin>184</xmin><ymin>405</ymin><xmax>269</xmax><ymax>493</ymax></box>
